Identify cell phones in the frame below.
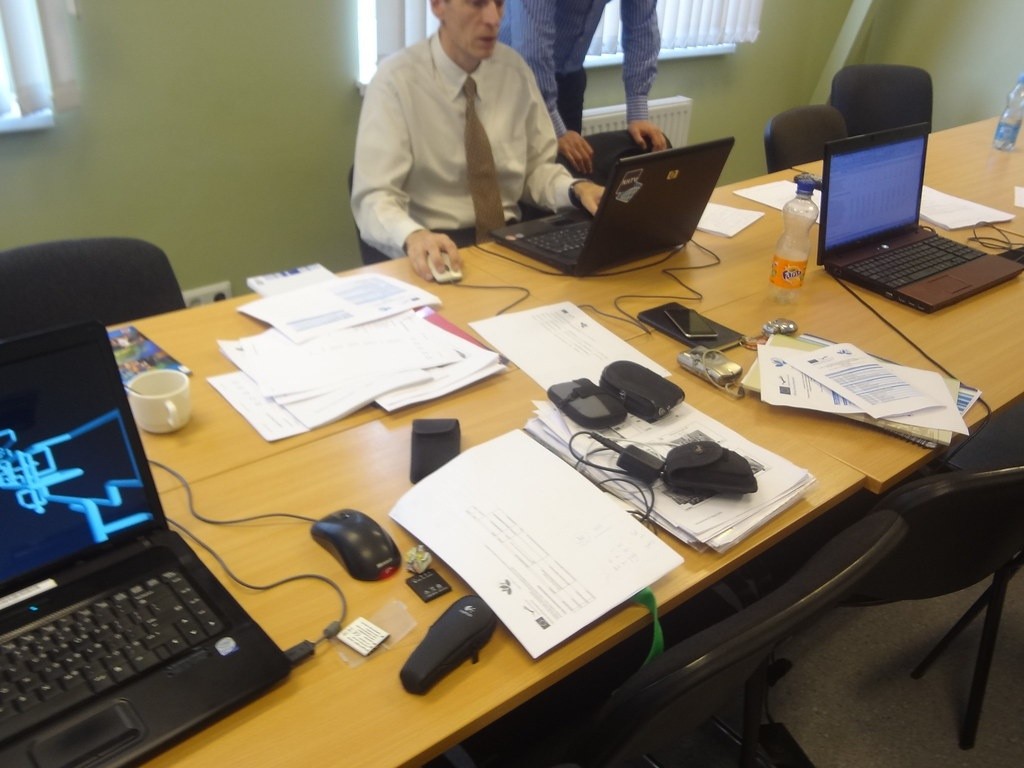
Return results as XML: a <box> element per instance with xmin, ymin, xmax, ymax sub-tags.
<box><xmin>664</xmin><ymin>308</ymin><xmax>719</xmax><ymax>339</ymax></box>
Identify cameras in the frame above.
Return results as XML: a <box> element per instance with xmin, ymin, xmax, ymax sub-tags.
<box><xmin>678</xmin><ymin>345</ymin><xmax>743</xmax><ymax>385</ymax></box>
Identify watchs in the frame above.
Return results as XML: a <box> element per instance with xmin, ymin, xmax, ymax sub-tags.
<box><xmin>568</xmin><ymin>180</ymin><xmax>583</xmax><ymax>208</ymax></box>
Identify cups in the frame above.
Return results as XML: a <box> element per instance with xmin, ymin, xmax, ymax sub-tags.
<box><xmin>125</xmin><ymin>370</ymin><xmax>192</xmax><ymax>433</ymax></box>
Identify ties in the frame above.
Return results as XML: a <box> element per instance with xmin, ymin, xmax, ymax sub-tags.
<box><xmin>462</xmin><ymin>78</ymin><xmax>506</xmax><ymax>245</ymax></box>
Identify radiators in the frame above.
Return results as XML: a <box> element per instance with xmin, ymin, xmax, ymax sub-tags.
<box><xmin>577</xmin><ymin>94</ymin><xmax>694</xmax><ymax>150</ymax></box>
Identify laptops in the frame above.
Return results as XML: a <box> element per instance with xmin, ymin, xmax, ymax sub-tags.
<box><xmin>817</xmin><ymin>122</ymin><xmax>1024</xmax><ymax>314</ymax></box>
<box><xmin>0</xmin><ymin>318</ymin><xmax>291</xmax><ymax>768</ymax></box>
<box><xmin>488</xmin><ymin>136</ymin><xmax>736</xmax><ymax>276</ymax></box>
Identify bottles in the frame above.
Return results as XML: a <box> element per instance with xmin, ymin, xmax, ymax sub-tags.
<box><xmin>993</xmin><ymin>75</ymin><xmax>1023</xmax><ymax>152</ymax></box>
<box><xmin>768</xmin><ymin>179</ymin><xmax>819</xmax><ymax>305</ymax></box>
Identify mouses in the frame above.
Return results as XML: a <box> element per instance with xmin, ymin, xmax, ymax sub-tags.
<box><xmin>310</xmin><ymin>509</ymin><xmax>402</xmax><ymax>583</ymax></box>
<box><xmin>425</xmin><ymin>251</ymin><xmax>462</xmax><ymax>283</ymax></box>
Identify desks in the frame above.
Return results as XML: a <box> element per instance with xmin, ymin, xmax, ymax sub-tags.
<box><xmin>106</xmin><ymin>116</ymin><xmax>1024</xmax><ymax>767</ymax></box>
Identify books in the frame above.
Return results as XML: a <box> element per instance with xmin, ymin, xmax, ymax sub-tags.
<box><xmin>207</xmin><ymin>263</ymin><xmax>982</xmax><ymax>659</ymax></box>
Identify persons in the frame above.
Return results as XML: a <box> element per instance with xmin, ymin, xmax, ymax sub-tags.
<box><xmin>497</xmin><ymin>0</ymin><xmax>667</xmax><ymax>174</ymax></box>
<box><xmin>350</xmin><ymin>0</ymin><xmax>608</xmax><ymax>281</ymax></box>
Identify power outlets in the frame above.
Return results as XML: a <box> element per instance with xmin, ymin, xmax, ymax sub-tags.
<box><xmin>182</xmin><ymin>279</ymin><xmax>233</xmax><ymax>308</ymax></box>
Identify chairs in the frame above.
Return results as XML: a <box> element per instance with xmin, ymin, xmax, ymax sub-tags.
<box><xmin>717</xmin><ymin>388</ymin><xmax>1024</xmax><ymax>753</ymax></box>
<box><xmin>438</xmin><ymin>505</ymin><xmax>912</xmax><ymax>768</ymax></box>
<box><xmin>0</xmin><ymin>235</ymin><xmax>187</xmax><ymax>340</ymax></box>
<box><xmin>348</xmin><ymin>62</ymin><xmax>936</xmax><ymax>262</ymax></box>
